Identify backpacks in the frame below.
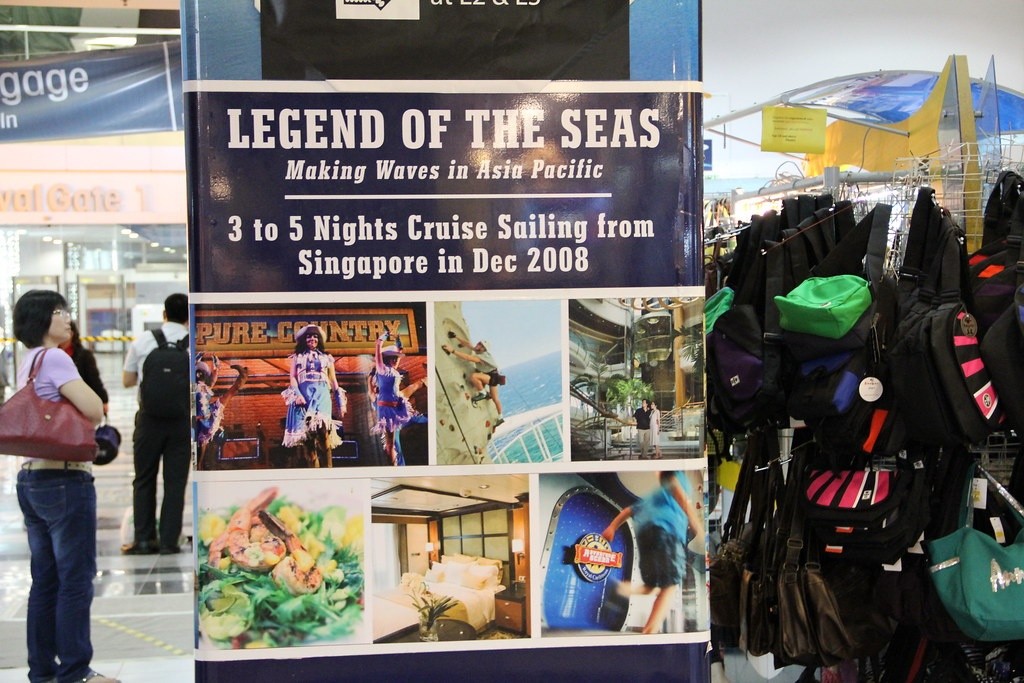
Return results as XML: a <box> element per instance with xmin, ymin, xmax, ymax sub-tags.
<box><xmin>139</xmin><ymin>327</ymin><xmax>191</xmax><ymax>420</ymax></box>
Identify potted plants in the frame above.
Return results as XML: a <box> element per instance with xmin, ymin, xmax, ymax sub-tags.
<box><xmin>605</xmin><ymin>375</ymin><xmax>656</xmax><ymax>440</ymax></box>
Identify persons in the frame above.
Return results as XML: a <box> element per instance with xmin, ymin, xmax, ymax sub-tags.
<box><xmin>12</xmin><ymin>290</ymin><xmax>122</xmax><ymax>683</ymax></box>
<box><xmin>196</xmin><ymin>352</ymin><xmax>249</xmax><ymax>471</ymax></box>
<box><xmin>602</xmin><ymin>470</ymin><xmax>703</xmax><ymax>634</ymax></box>
<box><xmin>442</xmin><ymin>331</ymin><xmax>505</xmax><ymax>427</ymax></box>
<box><xmin>281</xmin><ymin>325</ymin><xmax>347</xmax><ymax>468</ymax></box>
<box><xmin>57</xmin><ymin>319</ymin><xmax>108</xmax><ymax>414</ymax></box>
<box><xmin>632</xmin><ymin>399</ymin><xmax>663</xmax><ymax>460</ymax></box>
<box><xmin>122</xmin><ymin>294</ymin><xmax>189</xmax><ymax>553</ymax></box>
<box><xmin>368</xmin><ymin>319</ymin><xmax>428</xmax><ymax>467</ymax></box>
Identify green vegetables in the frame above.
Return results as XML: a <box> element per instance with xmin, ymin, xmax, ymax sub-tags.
<box><xmin>199</xmin><ymin>493</ymin><xmax>367</xmax><ymax>648</ymax></box>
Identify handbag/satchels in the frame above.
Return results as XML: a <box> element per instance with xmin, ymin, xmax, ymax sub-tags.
<box><xmin>0</xmin><ymin>345</ymin><xmax>100</xmax><ymax>463</ymax></box>
<box><xmin>702</xmin><ymin>171</ymin><xmax>1024</xmax><ymax>682</ymax></box>
<box><xmin>90</xmin><ymin>407</ymin><xmax>121</xmax><ymax>466</ymax></box>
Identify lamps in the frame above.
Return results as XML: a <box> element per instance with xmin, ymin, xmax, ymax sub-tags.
<box><xmin>425</xmin><ymin>542</ymin><xmax>436</xmax><ymax>557</ymax></box>
<box><xmin>511</xmin><ymin>539</ymin><xmax>525</xmax><ymax>565</ymax></box>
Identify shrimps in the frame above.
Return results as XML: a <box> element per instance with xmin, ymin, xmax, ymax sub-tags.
<box><xmin>225</xmin><ymin>488</ymin><xmax>285</xmax><ymax>572</ymax></box>
<box><xmin>258</xmin><ymin>509</ymin><xmax>321</xmax><ymax>597</ymax></box>
<box><xmin>209</xmin><ymin>517</ymin><xmax>281</xmax><ymax>569</ymax></box>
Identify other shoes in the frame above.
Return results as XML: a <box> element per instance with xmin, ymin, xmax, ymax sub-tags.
<box><xmin>120</xmin><ymin>540</ymin><xmax>155</xmax><ymax>554</ymax></box>
<box><xmin>69</xmin><ymin>668</ymin><xmax>122</xmax><ymax>683</ymax></box>
<box><xmin>158</xmin><ymin>542</ymin><xmax>180</xmax><ymax>554</ymax></box>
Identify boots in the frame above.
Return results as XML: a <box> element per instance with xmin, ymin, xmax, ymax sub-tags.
<box><xmin>230</xmin><ymin>364</ymin><xmax>253</xmax><ymax>391</ymax></box>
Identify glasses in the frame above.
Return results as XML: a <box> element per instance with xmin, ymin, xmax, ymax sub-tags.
<box><xmin>306</xmin><ymin>334</ymin><xmax>319</xmax><ymax>338</ymax></box>
<box><xmin>51</xmin><ymin>309</ymin><xmax>75</xmax><ymax>320</ymax></box>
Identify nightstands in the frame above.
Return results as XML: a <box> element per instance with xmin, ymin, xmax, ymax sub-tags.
<box><xmin>495</xmin><ymin>585</ymin><xmax>526</xmax><ymax>636</ymax></box>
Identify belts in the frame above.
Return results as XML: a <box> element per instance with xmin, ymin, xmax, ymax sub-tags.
<box><xmin>378</xmin><ymin>400</ymin><xmax>398</xmax><ymax>407</ymax></box>
<box><xmin>484</xmin><ymin>368</ymin><xmax>498</xmax><ymax>373</ymax></box>
<box><xmin>19</xmin><ymin>461</ymin><xmax>93</xmax><ymax>476</ymax></box>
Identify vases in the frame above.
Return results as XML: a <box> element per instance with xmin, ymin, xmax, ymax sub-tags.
<box><xmin>418</xmin><ymin>617</ymin><xmax>439</xmax><ymax>642</ymax></box>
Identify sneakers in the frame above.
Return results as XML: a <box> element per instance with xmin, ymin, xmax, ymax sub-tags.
<box><xmin>471</xmin><ymin>393</ymin><xmax>488</xmax><ymax>401</ymax></box>
<box><xmin>492</xmin><ymin>419</ymin><xmax>504</xmax><ymax>427</ymax></box>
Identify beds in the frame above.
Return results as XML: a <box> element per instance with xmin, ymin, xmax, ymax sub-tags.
<box><xmin>375</xmin><ymin>577</ymin><xmax>507</xmax><ymax>635</ymax></box>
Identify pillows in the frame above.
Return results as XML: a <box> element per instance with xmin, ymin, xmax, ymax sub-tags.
<box><xmin>424</xmin><ymin>552</ymin><xmax>503</xmax><ymax>589</ymax></box>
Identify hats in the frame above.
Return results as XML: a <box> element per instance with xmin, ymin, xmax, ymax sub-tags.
<box><xmin>479</xmin><ymin>340</ymin><xmax>489</xmax><ymax>351</ymax></box>
<box><xmin>380</xmin><ymin>345</ymin><xmax>406</xmax><ymax>357</ymax></box>
<box><xmin>294</xmin><ymin>323</ymin><xmax>327</xmax><ymax>344</ymax></box>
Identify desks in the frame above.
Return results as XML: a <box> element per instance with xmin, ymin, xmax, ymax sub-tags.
<box><xmin>372</xmin><ymin>596</ymin><xmax>422</xmax><ymax>644</ymax></box>
<box><xmin>388</xmin><ymin>618</ymin><xmax>477</xmax><ymax>643</ymax></box>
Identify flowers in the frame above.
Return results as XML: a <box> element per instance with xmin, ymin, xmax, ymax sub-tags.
<box><xmin>400</xmin><ymin>573</ymin><xmax>459</xmax><ymax>632</ymax></box>
